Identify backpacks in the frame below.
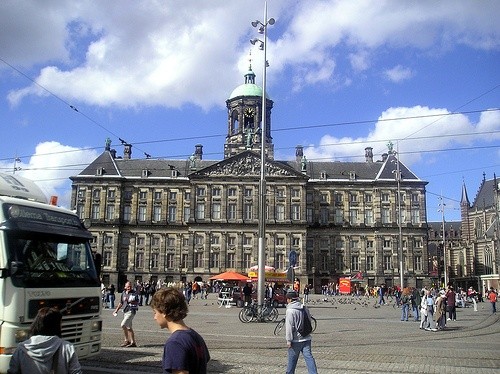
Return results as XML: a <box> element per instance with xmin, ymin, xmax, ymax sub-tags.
<box><xmin>293</xmin><ymin>306</ymin><xmax>312</xmax><ymax>338</ymax></box>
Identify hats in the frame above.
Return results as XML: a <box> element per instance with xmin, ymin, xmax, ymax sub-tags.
<box><xmin>286</xmin><ymin>292</ymin><xmax>298</xmax><ymax>300</ymax></box>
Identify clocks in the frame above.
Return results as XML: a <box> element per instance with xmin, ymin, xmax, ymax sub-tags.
<box><xmin>245</xmin><ymin>107</ymin><xmax>255</xmax><ymax>118</ymax></box>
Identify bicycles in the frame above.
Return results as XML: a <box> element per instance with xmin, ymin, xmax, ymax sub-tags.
<box><xmin>274</xmin><ymin>311</ymin><xmax>317</xmax><ymax>336</ymax></box>
<box><xmin>238</xmin><ymin>300</ymin><xmax>280</xmax><ymax>324</ymax></box>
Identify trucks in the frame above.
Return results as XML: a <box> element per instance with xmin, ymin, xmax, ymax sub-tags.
<box><xmin>1</xmin><ymin>171</ymin><xmax>104</xmax><ymax>374</ymax></box>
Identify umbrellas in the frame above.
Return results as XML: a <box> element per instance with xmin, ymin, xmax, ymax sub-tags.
<box><xmin>251</xmin><ymin>266</ymin><xmax>275</xmax><ymax>271</ymax></box>
<box><xmin>209</xmin><ymin>270</ymin><xmax>251</xmax><ymax>281</ymax></box>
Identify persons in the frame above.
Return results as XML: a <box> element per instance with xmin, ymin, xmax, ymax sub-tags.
<box><xmin>113</xmin><ymin>282</ymin><xmax>138</xmax><ymax>347</ymax></box>
<box><xmin>304</xmin><ymin>282</ymin><xmax>499</xmax><ymax>332</ymax></box>
<box><xmin>7</xmin><ymin>307</ymin><xmax>82</xmax><ymax>374</ymax></box>
<box><xmin>151</xmin><ymin>286</ymin><xmax>210</xmax><ymax>374</ymax></box>
<box><xmin>100</xmin><ymin>280</ymin><xmax>301</xmax><ymax>309</ymax></box>
<box><xmin>284</xmin><ymin>291</ymin><xmax>317</xmax><ymax>374</ymax></box>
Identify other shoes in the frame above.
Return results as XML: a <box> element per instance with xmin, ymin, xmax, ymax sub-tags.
<box><xmin>446</xmin><ymin>318</ymin><xmax>456</xmax><ymax>321</ymax></box>
<box><xmin>420</xmin><ymin>323</ymin><xmax>447</xmax><ymax>331</ymax></box>
<box><xmin>121</xmin><ymin>341</ymin><xmax>137</xmax><ymax>347</ymax></box>
